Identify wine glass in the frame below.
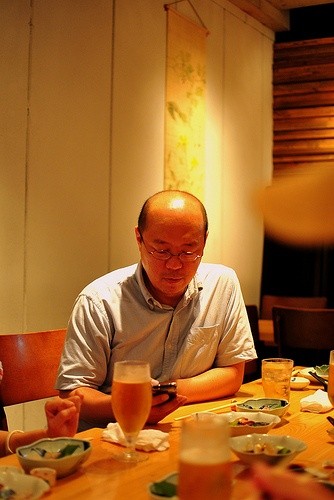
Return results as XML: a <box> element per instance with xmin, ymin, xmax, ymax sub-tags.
<box><xmin>111</xmin><ymin>359</ymin><xmax>152</xmax><ymax>463</ymax></box>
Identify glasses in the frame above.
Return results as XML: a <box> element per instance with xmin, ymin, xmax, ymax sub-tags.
<box><xmin>137</xmin><ymin>227</ymin><xmax>206</xmax><ymax>262</ymax></box>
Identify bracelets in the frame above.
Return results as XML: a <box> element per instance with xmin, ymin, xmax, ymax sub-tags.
<box><xmin>5</xmin><ymin>430</ymin><xmax>24</xmax><ymax>454</ymax></box>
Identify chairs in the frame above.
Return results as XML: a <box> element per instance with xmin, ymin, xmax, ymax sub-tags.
<box><xmin>0</xmin><ymin>329</ymin><xmax>66</xmax><ymax>430</ymax></box>
<box><xmin>242</xmin><ymin>295</ymin><xmax>334</xmax><ymax>384</ymax></box>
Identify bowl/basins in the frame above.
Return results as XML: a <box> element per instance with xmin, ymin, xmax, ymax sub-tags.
<box><xmin>290</xmin><ymin>377</ymin><xmax>310</xmax><ymax>389</ymax></box>
<box><xmin>300</xmin><ymin>368</ymin><xmax>328</xmax><ymax>382</ymax></box>
<box><xmin>0</xmin><ymin>471</ymin><xmax>50</xmax><ymax>500</ymax></box>
<box><xmin>15</xmin><ymin>437</ymin><xmax>92</xmax><ymax>479</ymax></box>
<box><xmin>235</xmin><ymin>399</ymin><xmax>290</xmax><ymax>419</ymax></box>
<box><xmin>216</xmin><ymin>412</ymin><xmax>281</xmax><ymax>436</ymax></box>
<box><xmin>229</xmin><ymin>433</ymin><xmax>307</xmax><ymax>465</ymax></box>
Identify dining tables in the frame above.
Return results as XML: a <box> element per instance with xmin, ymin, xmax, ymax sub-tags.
<box><xmin>0</xmin><ymin>366</ymin><xmax>334</xmax><ymax>500</ymax></box>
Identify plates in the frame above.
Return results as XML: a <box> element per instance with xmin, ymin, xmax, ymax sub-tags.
<box><xmin>146</xmin><ymin>473</ymin><xmax>237</xmax><ymax>500</ymax></box>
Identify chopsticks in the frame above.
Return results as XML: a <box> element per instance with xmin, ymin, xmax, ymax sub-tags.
<box><xmin>174</xmin><ymin>401</ymin><xmax>243</xmax><ymax>420</ymax></box>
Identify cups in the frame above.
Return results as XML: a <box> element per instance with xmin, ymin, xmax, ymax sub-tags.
<box><xmin>177</xmin><ymin>416</ymin><xmax>233</xmax><ymax>500</ymax></box>
<box><xmin>327</xmin><ymin>350</ymin><xmax>334</xmax><ymax>408</ymax></box>
<box><xmin>261</xmin><ymin>358</ymin><xmax>294</xmax><ymax>402</ymax></box>
<box><xmin>29</xmin><ymin>467</ymin><xmax>57</xmax><ymax>487</ymax></box>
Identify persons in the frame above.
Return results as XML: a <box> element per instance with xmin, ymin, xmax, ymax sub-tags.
<box><xmin>53</xmin><ymin>189</ymin><xmax>258</xmax><ymax>423</ymax></box>
<box><xmin>0</xmin><ymin>383</ymin><xmax>83</xmax><ymax>459</ymax></box>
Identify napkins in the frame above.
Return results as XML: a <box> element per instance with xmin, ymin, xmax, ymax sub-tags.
<box><xmin>301</xmin><ymin>390</ymin><xmax>333</xmax><ymax>413</ymax></box>
<box><xmin>102</xmin><ymin>422</ymin><xmax>168</xmax><ymax>452</ymax></box>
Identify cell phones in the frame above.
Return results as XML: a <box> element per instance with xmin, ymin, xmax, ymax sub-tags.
<box><xmin>152</xmin><ymin>381</ymin><xmax>177</xmax><ymax>404</ymax></box>
